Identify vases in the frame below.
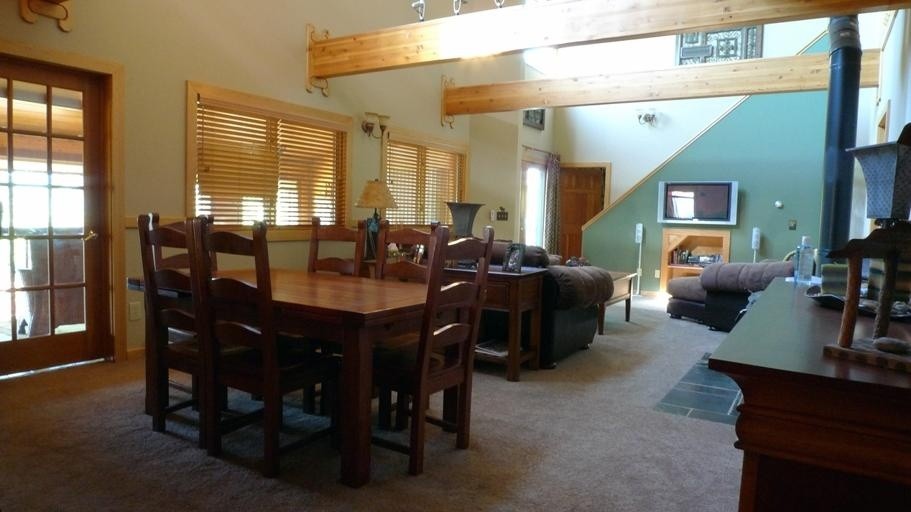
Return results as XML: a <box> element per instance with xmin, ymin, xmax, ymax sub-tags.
<box><xmin>446</xmin><ymin>199</ymin><xmax>486</xmax><ymax>270</ymax></box>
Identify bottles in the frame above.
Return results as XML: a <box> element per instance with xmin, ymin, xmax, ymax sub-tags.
<box><xmin>793</xmin><ymin>233</ymin><xmax>823</xmax><ymax>284</ymax></box>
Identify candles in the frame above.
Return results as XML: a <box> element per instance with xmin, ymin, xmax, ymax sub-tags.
<box><xmin>820</xmin><ymin>260</ymin><xmax>852</xmax><ymax>297</ymax></box>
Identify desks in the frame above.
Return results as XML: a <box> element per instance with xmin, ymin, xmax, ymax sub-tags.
<box><xmin>709</xmin><ymin>272</ymin><xmax>911</xmax><ymax>510</ymax></box>
<box><xmin>210</xmin><ymin>269</ymin><xmax>432</xmax><ymax>476</ymax></box>
<box><xmin>438</xmin><ymin>260</ymin><xmax>546</xmax><ymax>378</ymax></box>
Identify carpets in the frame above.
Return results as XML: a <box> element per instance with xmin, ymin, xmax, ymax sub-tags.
<box><xmin>652</xmin><ymin>349</ymin><xmax>744</xmax><ymax>430</ymax></box>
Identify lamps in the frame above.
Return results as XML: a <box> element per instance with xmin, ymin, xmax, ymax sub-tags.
<box><xmin>357</xmin><ymin>177</ymin><xmax>399</xmax><ymax>258</ymax></box>
<box><xmin>637</xmin><ymin>105</ymin><xmax>659</xmax><ymax>129</ymax></box>
<box><xmin>751</xmin><ymin>227</ymin><xmax>762</xmax><ymax>264</ymax></box>
<box><xmin>360</xmin><ymin>108</ymin><xmax>391</xmax><ymax>139</ymax></box>
<box><xmin>633</xmin><ymin>222</ymin><xmax>645</xmax><ymax>298</ymax></box>
<box><xmin>845</xmin><ymin>136</ymin><xmax>911</xmax><ymax>235</ymax></box>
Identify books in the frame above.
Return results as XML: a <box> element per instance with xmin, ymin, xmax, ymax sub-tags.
<box><xmin>673</xmin><ymin>248</ymin><xmax>689</xmax><ymax>264</ymax></box>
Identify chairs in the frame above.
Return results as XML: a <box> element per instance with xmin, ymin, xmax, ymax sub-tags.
<box><xmin>780</xmin><ymin>248</ymin><xmax>817</xmax><ymax>280</ymax></box>
<box><xmin>827</xmin><ymin>122</ymin><xmax>910</xmax><ymax>375</ymax></box>
<box><xmin>308</xmin><ymin>216</ymin><xmax>433</xmax><ymax>284</ymax></box>
<box><xmin>367</xmin><ymin>224</ymin><xmax>493</xmax><ymax>474</ymax></box>
<box><xmin>139</xmin><ymin>211</ymin><xmax>340</xmax><ymax>456</ymax></box>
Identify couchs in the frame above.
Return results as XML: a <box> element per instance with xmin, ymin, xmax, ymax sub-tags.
<box><xmin>434</xmin><ymin>233</ymin><xmax>613</xmax><ymax>371</ymax></box>
<box><xmin>666</xmin><ymin>257</ymin><xmax>794</xmax><ymax>330</ymax></box>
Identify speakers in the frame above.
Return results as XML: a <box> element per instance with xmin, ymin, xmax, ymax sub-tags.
<box><xmin>635</xmin><ymin>223</ymin><xmax>643</xmax><ymax>242</ymax></box>
<box><xmin>752</xmin><ymin>228</ymin><xmax>760</xmax><ymax>249</ymax></box>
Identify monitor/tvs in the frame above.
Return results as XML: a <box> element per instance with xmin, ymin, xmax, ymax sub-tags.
<box><xmin>657</xmin><ymin>180</ymin><xmax>738</xmax><ymax>225</ymax></box>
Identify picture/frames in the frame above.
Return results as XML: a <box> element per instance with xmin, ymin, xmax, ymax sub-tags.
<box><xmin>503</xmin><ymin>240</ymin><xmax>526</xmax><ymax>272</ymax></box>
<box><xmin>524</xmin><ymin>63</ymin><xmax>547</xmax><ymax>131</ymax></box>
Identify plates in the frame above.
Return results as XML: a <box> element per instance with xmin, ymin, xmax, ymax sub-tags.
<box><xmin>805</xmin><ymin>283</ymin><xmax>911</xmax><ymax>320</ymax></box>
